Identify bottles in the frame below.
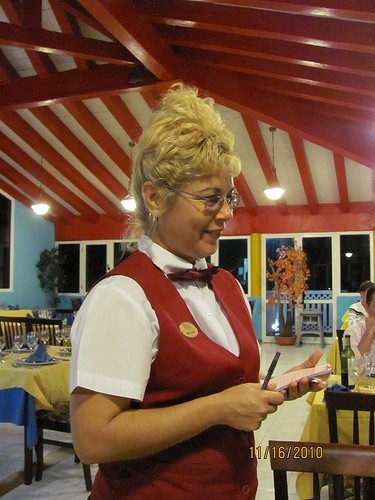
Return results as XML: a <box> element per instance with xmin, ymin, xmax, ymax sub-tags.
<box><xmin>340</xmin><ymin>335</ymin><xmax>355</xmax><ymax>389</ymax></box>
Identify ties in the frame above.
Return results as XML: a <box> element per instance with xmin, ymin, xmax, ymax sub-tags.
<box><xmin>167</xmin><ymin>265</ymin><xmax>221</xmax><ymax>283</ymax></box>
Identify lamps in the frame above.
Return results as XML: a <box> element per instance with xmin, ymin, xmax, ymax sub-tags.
<box><xmin>263</xmin><ymin>127</ymin><xmax>286</xmax><ymax>200</ymax></box>
<box><xmin>120</xmin><ymin>142</ymin><xmax>136</xmax><ymax>211</ymax></box>
<box><xmin>30</xmin><ymin>154</ymin><xmax>51</xmax><ymax>215</ymax></box>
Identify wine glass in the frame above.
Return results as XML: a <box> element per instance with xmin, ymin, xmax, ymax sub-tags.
<box><xmin>350</xmin><ymin>356</ymin><xmax>366</xmax><ymax>392</ymax></box>
<box><xmin>0</xmin><ymin>337</ymin><xmax>5</xmax><ymax>363</ymax></box>
<box><xmin>13</xmin><ymin>328</ymin><xmax>69</xmax><ymax>360</ymax></box>
<box><xmin>364</xmin><ymin>352</ymin><xmax>375</xmax><ymax>389</ymax></box>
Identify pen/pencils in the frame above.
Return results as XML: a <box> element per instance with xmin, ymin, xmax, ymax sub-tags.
<box><xmin>261</xmin><ymin>352</ymin><xmax>281</xmax><ymax>390</ymax></box>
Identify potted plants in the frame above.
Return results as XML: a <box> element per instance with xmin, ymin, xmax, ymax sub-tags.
<box><xmin>263</xmin><ymin>245</ymin><xmax>311</xmax><ymax>346</ymax></box>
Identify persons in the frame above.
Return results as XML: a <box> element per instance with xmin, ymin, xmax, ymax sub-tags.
<box><xmin>343</xmin><ymin>286</ymin><xmax>375</xmax><ymax>378</ymax></box>
<box><xmin>69</xmin><ymin>83</ymin><xmax>325</xmax><ymax>500</ymax></box>
<box><xmin>348</xmin><ymin>281</ymin><xmax>375</xmax><ymax>326</ymax></box>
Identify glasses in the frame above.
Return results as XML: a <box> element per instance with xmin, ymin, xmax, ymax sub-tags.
<box><xmin>160</xmin><ymin>184</ymin><xmax>242</xmax><ymax>211</ymax></box>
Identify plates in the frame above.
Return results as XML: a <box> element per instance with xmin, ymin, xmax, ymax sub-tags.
<box><xmin>13</xmin><ymin>348</ymin><xmax>35</xmax><ymax>352</ymax></box>
<box><xmin>16</xmin><ymin>358</ymin><xmax>62</xmax><ymax>365</ymax></box>
<box><xmin>57</xmin><ymin>348</ymin><xmax>71</xmax><ymax>355</ymax></box>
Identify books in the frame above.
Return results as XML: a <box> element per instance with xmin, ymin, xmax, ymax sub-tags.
<box><xmin>261</xmin><ymin>363</ymin><xmax>331</xmax><ymax>391</ymax></box>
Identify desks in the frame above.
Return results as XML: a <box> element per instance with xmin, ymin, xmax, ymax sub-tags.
<box><xmin>0</xmin><ymin>344</ymin><xmax>73</xmax><ymax>485</ymax></box>
<box><xmin>295</xmin><ymin>311</ymin><xmax>325</xmax><ymax>348</ymax></box>
<box><xmin>294</xmin><ymin>375</ymin><xmax>375</xmax><ymax>500</ymax></box>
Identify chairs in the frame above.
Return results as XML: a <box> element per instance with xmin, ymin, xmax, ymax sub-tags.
<box><xmin>268</xmin><ymin>440</ymin><xmax>375</xmax><ymax>500</ymax></box>
<box><xmin>0</xmin><ymin>315</ymin><xmax>64</xmax><ymax>350</ymax></box>
<box><xmin>324</xmin><ymin>390</ymin><xmax>374</xmax><ymax>500</ymax></box>
<box><xmin>33</xmin><ymin>401</ymin><xmax>93</xmax><ymax>493</ymax></box>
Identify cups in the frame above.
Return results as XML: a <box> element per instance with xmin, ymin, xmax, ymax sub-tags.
<box><xmin>38</xmin><ymin>310</ymin><xmax>52</xmax><ymax>319</ymax></box>
